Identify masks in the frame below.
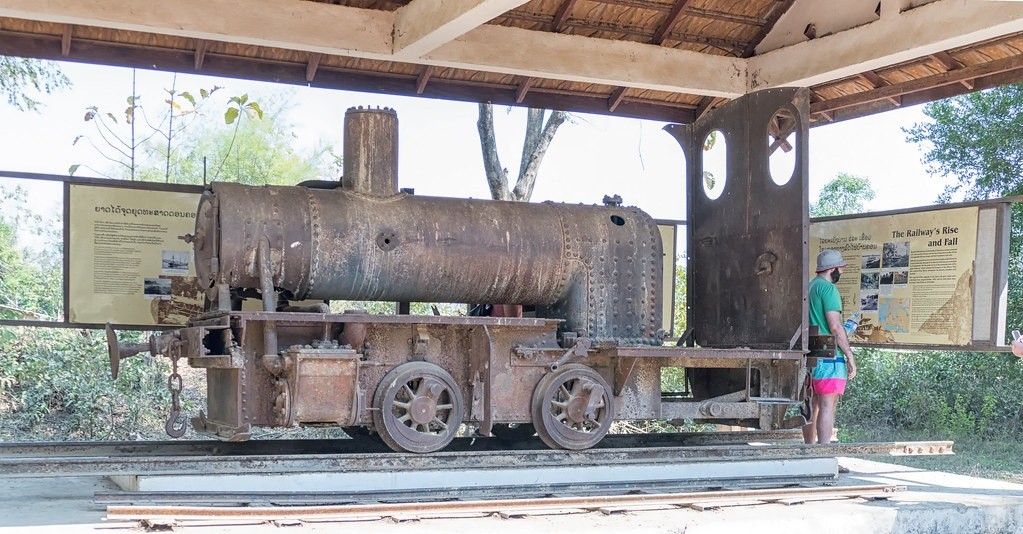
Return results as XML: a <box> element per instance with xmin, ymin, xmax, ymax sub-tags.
<box><xmin>830</xmin><ymin>268</ymin><xmax>841</xmax><ymax>284</ymax></box>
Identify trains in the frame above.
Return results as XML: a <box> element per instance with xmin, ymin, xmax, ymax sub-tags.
<box><xmin>104</xmin><ymin>86</ymin><xmax>838</xmax><ymax>452</ymax></box>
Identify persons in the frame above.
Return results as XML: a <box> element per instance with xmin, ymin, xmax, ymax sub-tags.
<box><xmin>799</xmin><ymin>247</ymin><xmax>857</xmax><ymax>474</ymax></box>
<box><xmin>1011</xmin><ymin>335</ymin><xmax>1023</xmax><ymax>357</ymax></box>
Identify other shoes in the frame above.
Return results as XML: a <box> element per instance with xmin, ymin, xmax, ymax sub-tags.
<box><xmin>838</xmin><ymin>465</ymin><xmax>849</xmax><ymax>473</ymax></box>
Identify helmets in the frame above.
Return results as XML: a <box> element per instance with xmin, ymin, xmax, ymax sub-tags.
<box><xmin>814</xmin><ymin>249</ymin><xmax>848</xmax><ymax>273</ymax></box>
